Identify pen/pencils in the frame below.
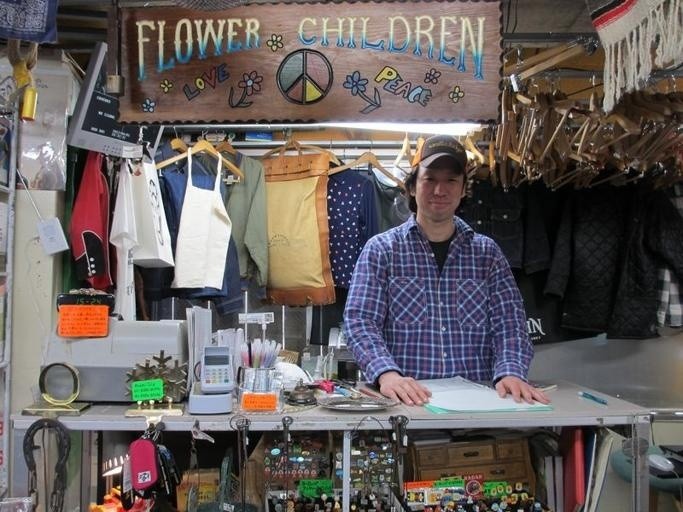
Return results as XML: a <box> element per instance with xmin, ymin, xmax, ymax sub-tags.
<box><xmin>578</xmin><ymin>390</ymin><xmax>608</xmax><ymax>404</ymax></box>
<box><xmin>241</xmin><ymin>339</ymin><xmax>282</xmax><ymax>369</ymax></box>
<box><xmin>359</xmin><ymin>388</ymin><xmax>378</xmax><ymax>398</ymax></box>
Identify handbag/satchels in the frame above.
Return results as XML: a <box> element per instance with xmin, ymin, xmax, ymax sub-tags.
<box><xmin>128</xmin><ymin>158</ymin><xmax>175</xmax><ymax>269</ymax></box>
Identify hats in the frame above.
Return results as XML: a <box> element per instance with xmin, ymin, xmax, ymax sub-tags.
<box><xmin>411</xmin><ymin>133</ymin><xmax>468</xmax><ymax>169</ymax></box>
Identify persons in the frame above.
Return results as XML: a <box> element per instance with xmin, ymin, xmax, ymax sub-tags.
<box><xmin>341</xmin><ymin>136</ymin><xmax>552</xmax><ymax>407</ymax></box>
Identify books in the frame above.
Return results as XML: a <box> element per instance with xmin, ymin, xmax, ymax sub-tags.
<box><xmin>526</xmin><ymin>424</ymin><xmax>615</xmax><ymax>511</ymax></box>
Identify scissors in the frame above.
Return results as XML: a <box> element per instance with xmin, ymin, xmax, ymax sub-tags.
<box><xmin>330</xmin><ymin>378</ymin><xmax>360</xmax><ymax>394</ymax></box>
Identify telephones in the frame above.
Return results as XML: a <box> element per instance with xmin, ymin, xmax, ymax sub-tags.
<box><xmin>328</xmin><ymin>327</ymin><xmax>347</xmax><ymax>350</ymax></box>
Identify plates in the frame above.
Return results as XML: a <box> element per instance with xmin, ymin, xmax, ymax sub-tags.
<box><xmin>317</xmin><ymin>395</ymin><xmax>400</xmax><ymax>412</ymax></box>
